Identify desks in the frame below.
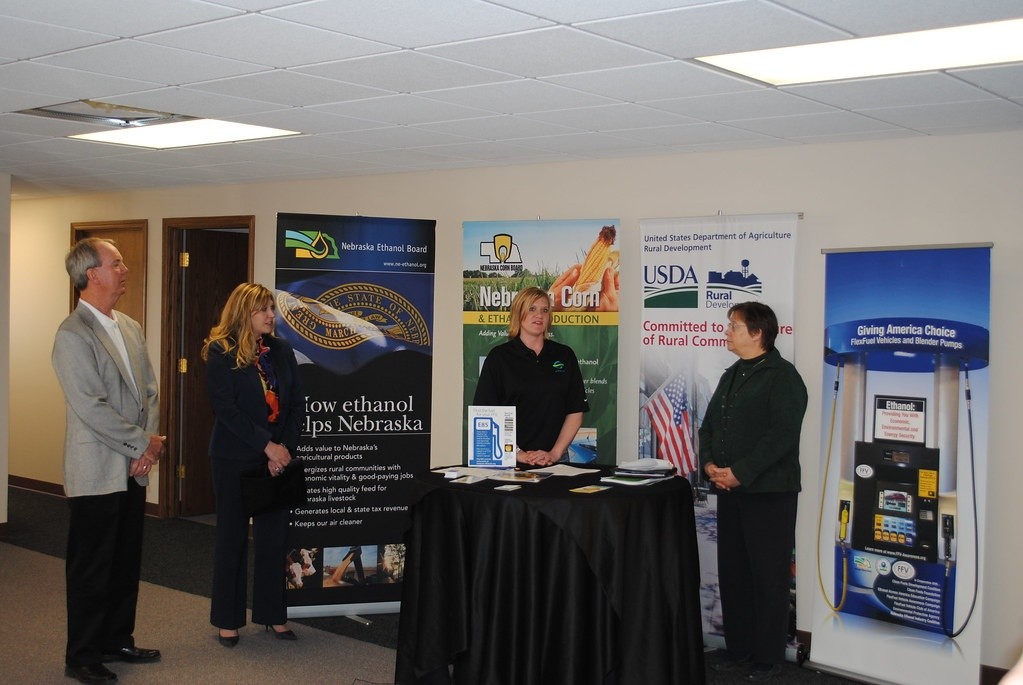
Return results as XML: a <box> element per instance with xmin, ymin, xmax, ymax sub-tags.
<box><xmin>395</xmin><ymin>464</ymin><xmax>706</xmax><ymax>685</ymax></box>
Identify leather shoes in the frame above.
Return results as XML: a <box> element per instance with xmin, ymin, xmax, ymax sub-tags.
<box><xmin>64</xmin><ymin>661</ymin><xmax>118</xmax><ymax>685</ymax></box>
<box><xmin>102</xmin><ymin>644</ymin><xmax>161</xmax><ymax>664</ymax></box>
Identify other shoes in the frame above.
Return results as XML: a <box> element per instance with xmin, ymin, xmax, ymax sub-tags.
<box><xmin>711</xmin><ymin>655</ymin><xmax>755</xmax><ymax>671</ymax></box>
<box><xmin>744</xmin><ymin>661</ymin><xmax>783</xmax><ymax>682</ymax></box>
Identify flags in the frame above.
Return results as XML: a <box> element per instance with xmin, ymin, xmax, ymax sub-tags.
<box><xmin>643</xmin><ymin>367</ymin><xmax>698</xmax><ymax>478</ymax></box>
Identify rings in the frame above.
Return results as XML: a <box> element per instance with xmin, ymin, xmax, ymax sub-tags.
<box><xmin>276</xmin><ymin>467</ymin><xmax>279</xmax><ymax>470</ymax></box>
<box><xmin>144</xmin><ymin>465</ymin><xmax>147</xmax><ymax>468</ymax></box>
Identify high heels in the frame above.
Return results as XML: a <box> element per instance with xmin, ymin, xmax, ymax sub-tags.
<box><xmin>219</xmin><ymin>628</ymin><xmax>239</xmax><ymax>649</ymax></box>
<box><xmin>265</xmin><ymin>624</ymin><xmax>299</xmax><ymax>640</ymax></box>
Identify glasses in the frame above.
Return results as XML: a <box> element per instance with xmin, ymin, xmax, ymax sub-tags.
<box><xmin>728</xmin><ymin>322</ymin><xmax>746</xmax><ymax>331</ymax></box>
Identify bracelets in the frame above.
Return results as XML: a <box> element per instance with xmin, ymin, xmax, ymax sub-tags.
<box><xmin>516</xmin><ymin>447</ymin><xmax>521</xmax><ymax>454</ymax></box>
<box><xmin>280</xmin><ymin>443</ymin><xmax>286</xmax><ymax>447</ymax></box>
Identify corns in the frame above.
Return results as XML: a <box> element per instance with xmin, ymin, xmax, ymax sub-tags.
<box><xmin>562</xmin><ymin>225</ymin><xmax>618</xmax><ymax>310</ymax></box>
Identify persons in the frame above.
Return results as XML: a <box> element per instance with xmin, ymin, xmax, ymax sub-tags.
<box><xmin>473</xmin><ymin>286</ymin><xmax>590</xmax><ymax>466</ymax></box>
<box><xmin>547</xmin><ymin>263</ymin><xmax>620</xmax><ymax>313</ymax></box>
<box><xmin>697</xmin><ymin>300</ymin><xmax>809</xmax><ymax>685</ymax></box>
<box><xmin>200</xmin><ymin>284</ymin><xmax>305</xmax><ymax>646</ymax></box>
<box><xmin>51</xmin><ymin>235</ymin><xmax>168</xmax><ymax>685</ymax></box>
